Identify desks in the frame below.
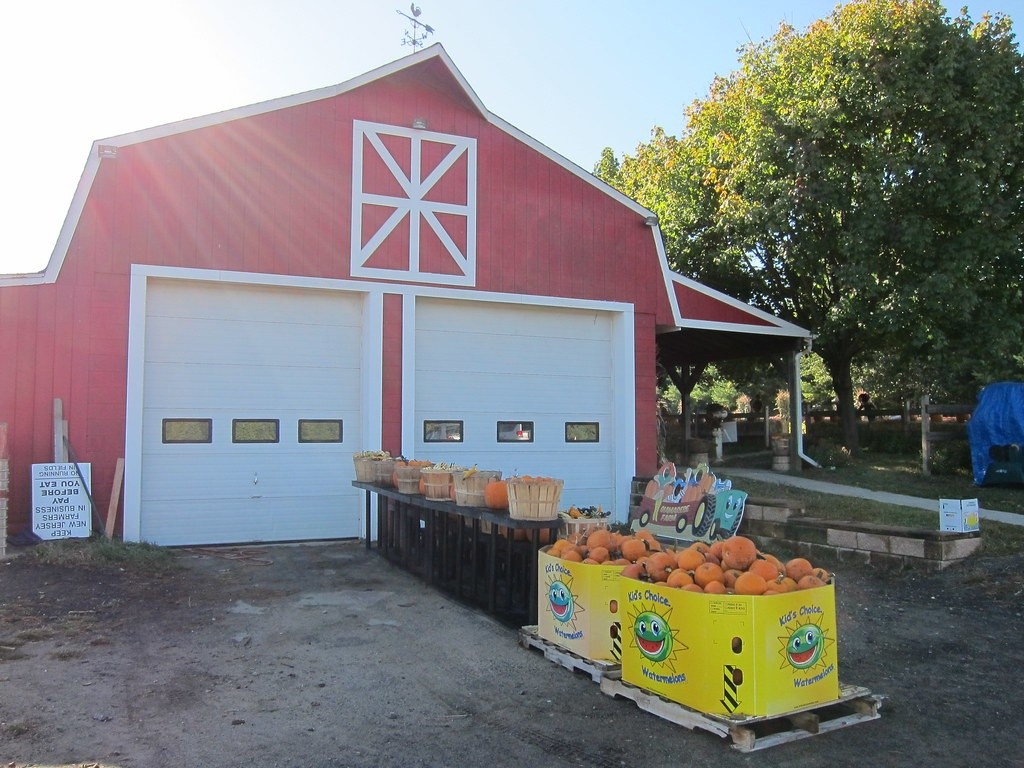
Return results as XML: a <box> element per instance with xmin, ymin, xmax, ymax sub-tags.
<box><xmin>352</xmin><ymin>480</ymin><xmax>564</xmax><ymax>630</ymax></box>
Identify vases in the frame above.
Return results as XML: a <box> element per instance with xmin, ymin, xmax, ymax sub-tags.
<box><xmin>688</xmin><ymin>437</ymin><xmax>711</xmax><ymax>452</ymax></box>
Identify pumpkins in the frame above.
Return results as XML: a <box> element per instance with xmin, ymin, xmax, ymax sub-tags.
<box><xmin>393</xmin><ymin>458</ymin><xmax>510</xmax><ymax>509</ymax></box>
<box><xmin>546</xmin><ymin>530</ymin><xmax>836</xmax><ymax>595</ymax></box>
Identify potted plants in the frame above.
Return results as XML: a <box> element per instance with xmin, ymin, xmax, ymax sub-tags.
<box><xmin>770</xmin><ymin>433</ymin><xmax>790</xmax><ymax>455</ymax></box>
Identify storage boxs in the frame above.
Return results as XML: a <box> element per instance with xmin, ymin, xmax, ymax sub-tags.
<box><xmin>939</xmin><ymin>499</ymin><xmax>979</xmax><ymax>533</ymax></box>
<box><xmin>538</xmin><ymin>545</ymin><xmax>838</xmax><ymax>716</ymax></box>
